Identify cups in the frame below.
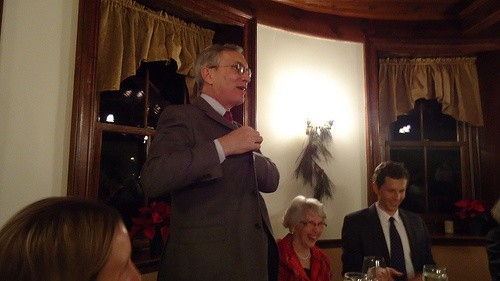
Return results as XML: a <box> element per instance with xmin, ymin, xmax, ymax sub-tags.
<box><xmin>362</xmin><ymin>257</ymin><xmax>384</xmax><ymax>281</ymax></box>
<box><xmin>422</xmin><ymin>264</ymin><xmax>448</xmax><ymax>281</ymax></box>
<box><xmin>345</xmin><ymin>273</ymin><xmax>366</xmax><ymax>281</ymax></box>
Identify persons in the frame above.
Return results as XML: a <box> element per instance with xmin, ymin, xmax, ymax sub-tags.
<box><xmin>141</xmin><ymin>42</ymin><xmax>281</xmax><ymax>280</ymax></box>
<box><xmin>340</xmin><ymin>160</ymin><xmax>437</xmax><ymax>281</ymax></box>
<box><xmin>274</xmin><ymin>194</ymin><xmax>335</xmax><ymax>281</ymax></box>
<box><xmin>0</xmin><ymin>195</ymin><xmax>142</xmax><ymax>280</ymax></box>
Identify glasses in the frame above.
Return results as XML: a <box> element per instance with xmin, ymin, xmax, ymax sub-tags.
<box><xmin>300</xmin><ymin>220</ymin><xmax>328</xmax><ymax>231</ymax></box>
<box><xmin>208</xmin><ymin>62</ymin><xmax>252</xmax><ymax>78</ymax></box>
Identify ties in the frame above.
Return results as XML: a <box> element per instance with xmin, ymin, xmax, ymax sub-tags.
<box><xmin>389</xmin><ymin>217</ymin><xmax>408</xmax><ymax>281</ymax></box>
<box><xmin>224</xmin><ymin>111</ymin><xmax>233</xmax><ymax>124</ymax></box>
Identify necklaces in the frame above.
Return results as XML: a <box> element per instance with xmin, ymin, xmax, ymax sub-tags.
<box><xmin>295</xmin><ymin>248</ymin><xmax>311</xmax><ymax>261</ymax></box>
